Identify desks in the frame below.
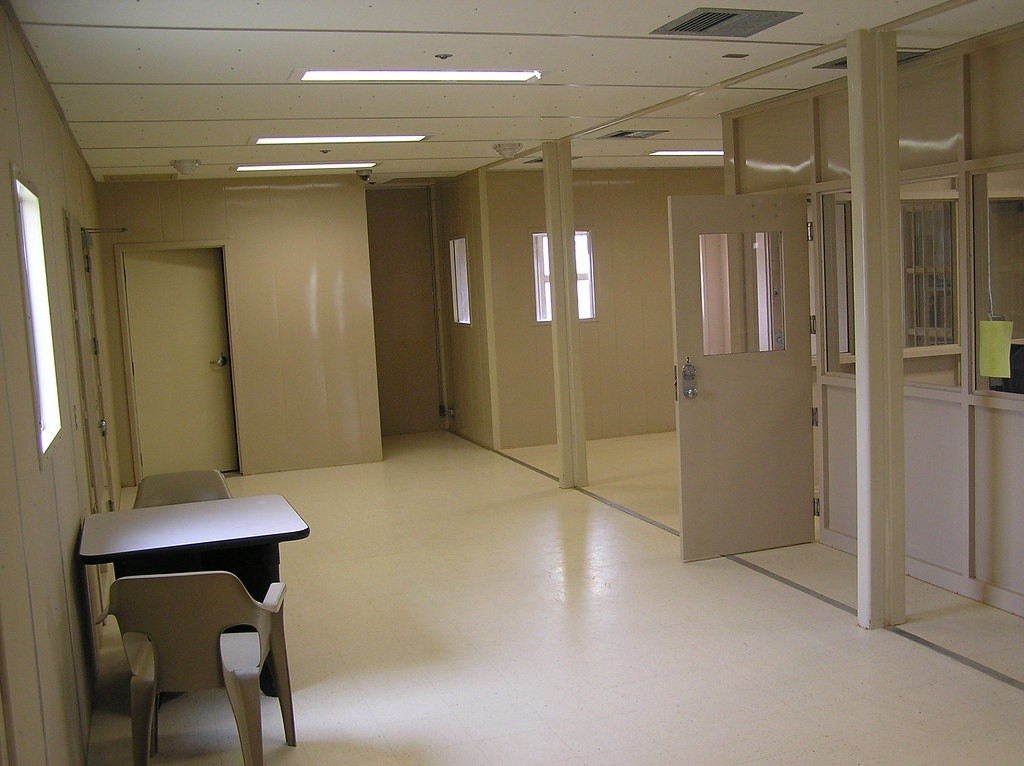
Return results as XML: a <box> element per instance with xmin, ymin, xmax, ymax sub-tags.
<box><xmin>80</xmin><ymin>493</ymin><xmax>310</xmax><ymax>698</ymax></box>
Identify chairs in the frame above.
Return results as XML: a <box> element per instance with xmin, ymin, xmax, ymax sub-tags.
<box><xmin>96</xmin><ymin>570</ymin><xmax>297</xmax><ymax>766</ymax></box>
<box><xmin>133</xmin><ymin>469</ymin><xmax>232</xmax><ymax>509</ymax></box>
